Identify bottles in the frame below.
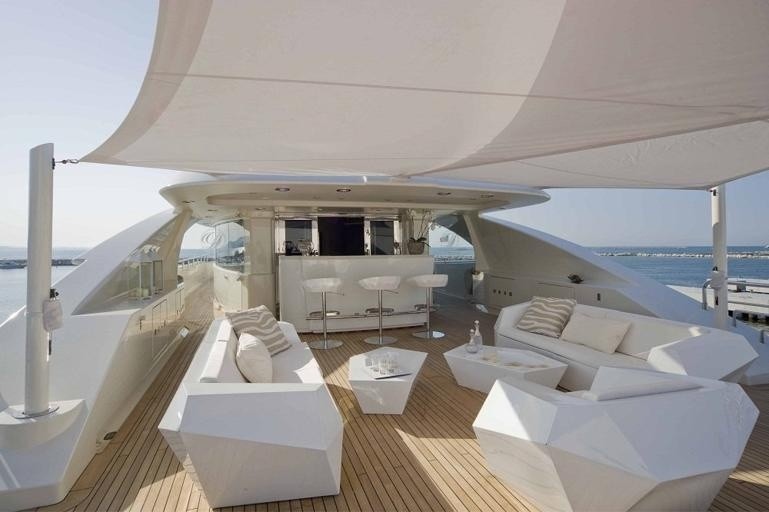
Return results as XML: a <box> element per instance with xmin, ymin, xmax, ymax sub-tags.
<box><xmin>473</xmin><ymin>320</ymin><xmax>482</xmax><ymax>349</ymax></box>
<box><xmin>465</xmin><ymin>329</ymin><xmax>478</xmax><ymax>353</ymax></box>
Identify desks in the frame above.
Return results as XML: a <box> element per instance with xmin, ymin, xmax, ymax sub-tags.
<box><xmin>277</xmin><ymin>256</ymin><xmax>433</xmax><ymax>333</ymax></box>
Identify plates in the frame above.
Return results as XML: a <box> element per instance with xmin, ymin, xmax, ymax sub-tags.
<box><xmin>364</xmin><ymin>363</ymin><xmax>413</xmax><ymax>380</ymax></box>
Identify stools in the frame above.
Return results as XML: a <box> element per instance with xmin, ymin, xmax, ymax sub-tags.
<box><xmin>302</xmin><ymin>279</ymin><xmax>345</xmax><ymax>350</ymax></box>
<box><xmin>407</xmin><ymin>274</ymin><xmax>448</xmax><ymax>339</ymax></box>
<box><xmin>358</xmin><ymin>276</ymin><xmax>401</xmax><ymax>346</ymax></box>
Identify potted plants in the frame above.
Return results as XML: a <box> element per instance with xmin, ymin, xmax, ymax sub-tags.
<box><xmin>407</xmin><ymin>237</ymin><xmax>430</xmax><ymax>255</ymax></box>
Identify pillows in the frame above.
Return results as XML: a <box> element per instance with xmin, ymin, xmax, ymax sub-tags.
<box><xmin>561</xmin><ymin>313</ymin><xmax>632</xmax><ymax>354</ymax></box>
<box><xmin>225</xmin><ymin>304</ymin><xmax>291</xmax><ymax>357</ymax></box>
<box><xmin>236</xmin><ymin>333</ymin><xmax>272</xmax><ymax>383</ymax></box>
<box><xmin>514</xmin><ymin>297</ymin><xmax>577</xmax><ymax>337</ymax></box>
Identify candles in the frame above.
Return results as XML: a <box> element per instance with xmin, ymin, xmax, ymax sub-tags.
<box><xmin>137</xmin><ymin>288</ymin><xmax>148</xmax><ymax>297</ymax></box>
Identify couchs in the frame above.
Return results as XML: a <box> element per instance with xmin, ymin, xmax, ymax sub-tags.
<box><xmin>156</xmin><ymin>319</ymin><xmax>344</xmax><ymax>509</ymax></box>
<box><xmin>493</xmin><ymin>301</ymin><xmax>758</xmax><ymax>392</ymax></box>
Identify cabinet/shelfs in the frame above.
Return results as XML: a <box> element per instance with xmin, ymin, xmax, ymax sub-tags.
<box><xmin>152</xmin><ymin>299</ymin><xmax>169</xmax><ymax>361</ymax></box>
<box><xmin>175</xmin><ymin>288</ymin><xmax>186</xmax><ymax>332</ymax></box>
<box><xmin>489</xmin><ymin>277</ymin><xmax>575</xmax><ymax>316</ymax></box>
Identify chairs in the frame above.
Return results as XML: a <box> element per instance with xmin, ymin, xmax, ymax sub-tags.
<box><xmin>472</xmin><ymin>364</ymin><xmax>759</xmax><ymax>512</ymax></box>
